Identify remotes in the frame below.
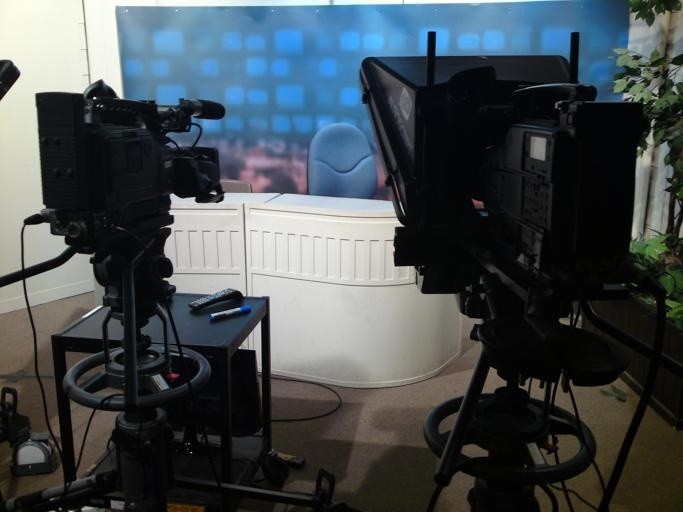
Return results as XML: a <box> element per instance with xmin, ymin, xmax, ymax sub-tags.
<box><xmin>188</xmin><ymin>288</ymin><xmax>243</xmax><ymax>310</ymax></box>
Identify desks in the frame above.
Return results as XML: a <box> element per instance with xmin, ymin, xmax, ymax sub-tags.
<box><xmin>47</xmin><ymin>293</ymin><xmax>276</xmax><ymax>511</ymax></box>
<box><xmin>159</xmin><ymin>191</ymin><xmax>463</xmax><ymax>391</ymax></box>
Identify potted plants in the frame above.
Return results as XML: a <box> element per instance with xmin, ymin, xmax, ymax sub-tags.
<box><xmin>580</xmin><ymin>1</ymin><xmax>682</xmax><ymax>427</ymax></box>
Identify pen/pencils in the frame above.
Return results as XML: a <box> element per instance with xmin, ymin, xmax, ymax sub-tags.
<box><xmin>206</xmin><ymin>304</ymin><xmax>252</xmax><ymax>320</ymax></box>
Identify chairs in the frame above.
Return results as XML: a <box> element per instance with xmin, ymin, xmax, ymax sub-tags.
<box><xmin>304</xmin><ymin>121</ymin><xmax>378</xmax><ymax>200</ymax></box>
